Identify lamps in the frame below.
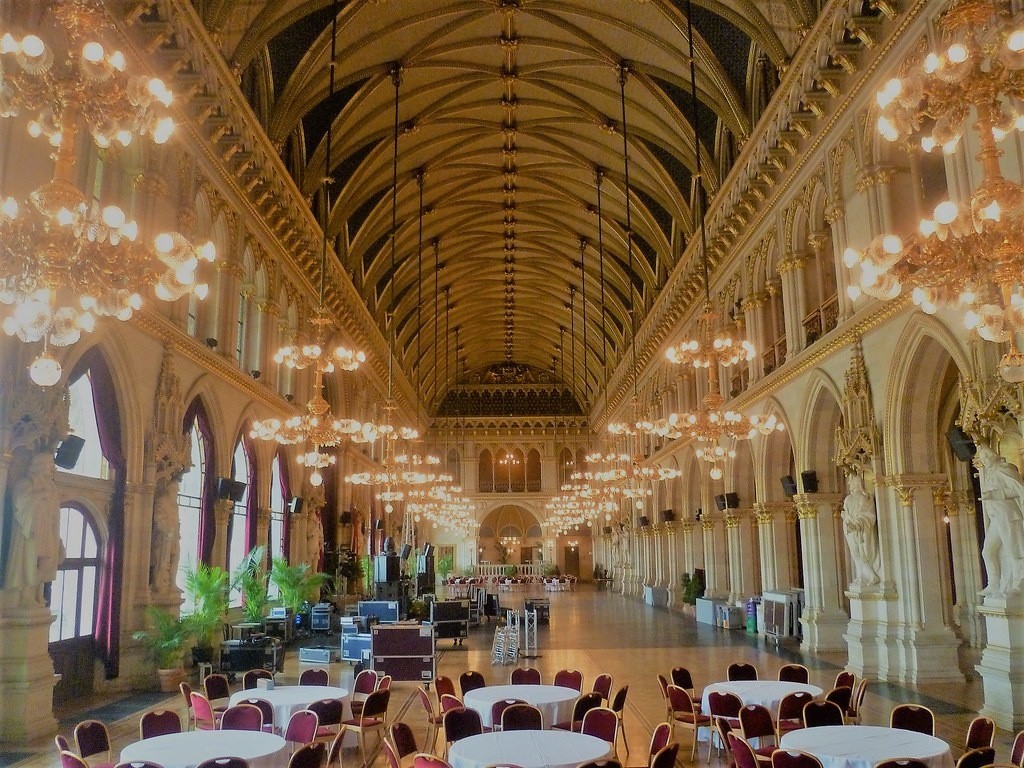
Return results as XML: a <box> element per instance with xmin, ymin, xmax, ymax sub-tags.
<box><xmin>250</xmin><ymin>306</ymin><xmax>393</xmax><ymax>486</ymax></box>
<box><xmin>541</xmin><ymin>394</ymin><xmax>682</xmax><ymax>539</ymax></box>
<box><xmin>653</xmin><ymin>299</ymin><xmax>785</xmax><ymax>483</ymax></box>
<box><xmin>0</xmin><ymin>0</ymin><xmax>214</xmax><ymax>388</ymax></box>
<box><xmin>342</xmin><ymin>399</ymin><xmax>482</xmax><ymax>539</ymax></box>
<box><xmin>844</xmin><ymin>0</ymin><xmax>1024</xmax><ymax>384</ymax></box>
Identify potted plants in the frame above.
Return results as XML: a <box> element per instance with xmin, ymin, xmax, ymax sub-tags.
<box><xmin>132</xmin><ymin>604</ymin><xmax>220</xmax><ymax>692</ymax></box>
<box><xmin>179</xmin><ymin>559</ymin><xmax>236</xmax><ymax>666</ymax></box>
<box><xmin>438</xmin><ymin>554</ymin><xmax>453</xmax><ymax>585</ymax></box>
<box><xmin>679</xmin><ymin>572</ymin><xmax>703</xmax><ymax>617</ymax></box>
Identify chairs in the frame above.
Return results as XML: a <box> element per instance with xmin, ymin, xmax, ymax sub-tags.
<box><xmin>492</xmin><ymin>576</ymin><xmax>537</xmax><ymax>593</ymax></box>
<box><xmin>446</xmin><ymin>575</ymin><xmax>489</xmax><ymax>594</ymax></box>
<box><xmin>53</xmin><ymin>668</ymin><xmax>1024</xmax><ymax>768</ymax></box>
<box><xmin>222</xmin><ymin>622</ymin><xmax>234</xmax><ymax>640</ymax></box>
<box><xmin>540</xmin><ymin>574</ymin><xmax>578</xmax><ymax>592</ymax></box>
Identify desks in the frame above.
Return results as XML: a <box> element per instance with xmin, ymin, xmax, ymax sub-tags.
<box><xmin>552</xmin><ymin>580</ymin><xmax>570</xmax><ymax>591</ymax></box>
<box><xmin>697</xmin><ymin>680</ymin><xmax>824</xmax><ymax>752</ymax></box>
<box><xmin>779</xmin><ymin>724</ymin><xmax>955</xmax><ymax>768</ymax></box>
<box><xmin>448</xmin><ymin>729</ymin><xmax>611</xmax><ymax>768</ymax></box>
<box><xmin>464</xmin><ymin>684</ymin><xmax>581</xmax><ymax>733</ymax></box>
<box><xmin>228</xmin><ymin>685</ymin><xmax>358</xmax><ymax>762</ymax></box>
<box><xmin>119</xmin><ymin>729</ymin><xmax>290</xmax><ymax>768</ymax></box>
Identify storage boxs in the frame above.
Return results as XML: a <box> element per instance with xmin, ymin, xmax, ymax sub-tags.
<box><xmin>299</xmin><ymin>646</ymin><xmax>342</xmax><ymax>664</ymax></box>
<box><xmin>219</xmin><ymin>640</ymin><xmax>289</xmax><ymax>674</ymax></box>
<box><xmin>265</xmin><ymin>603</ymin><xmax>336</xmax><ymax>645</ymax></box>
<box><xmin>340</xmin><ymin>598</ymin><xmax>471</xmax><ymax>690</ymax></box>
<box><xmin>444</xmin><ymin>584</ymin><xmax>550</xmax><ymax>626</ymax></box>
